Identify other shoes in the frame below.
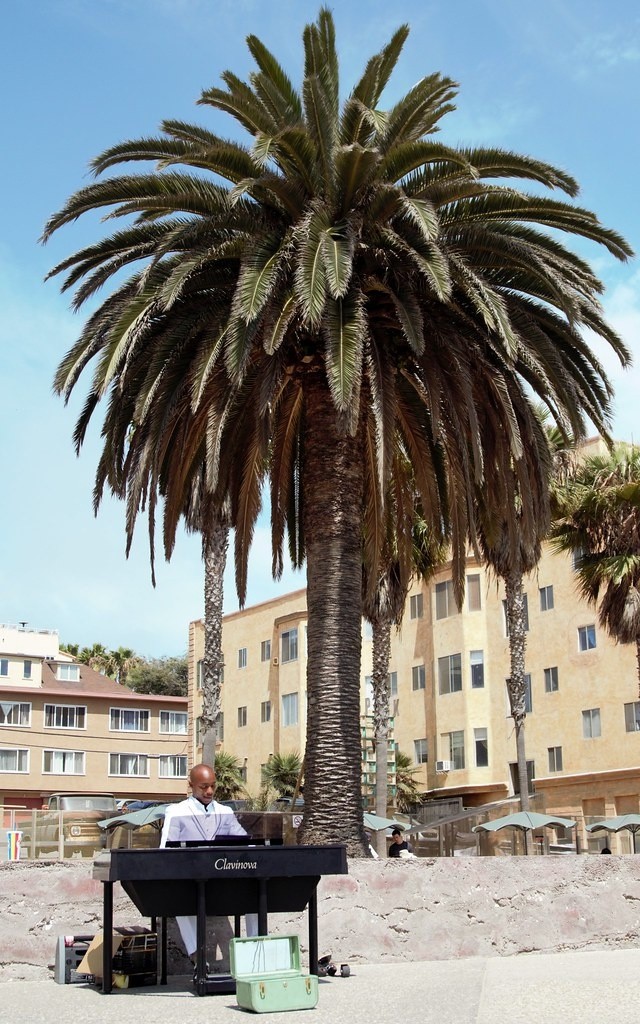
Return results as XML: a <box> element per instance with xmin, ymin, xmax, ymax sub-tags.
<box><xmin>192</xmin><ymin>964</ymin><xmax>208</xmax><ymax>985</ymax></box>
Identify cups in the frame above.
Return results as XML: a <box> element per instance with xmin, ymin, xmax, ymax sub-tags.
<box><xmin>6</xmin><ymin>831</ymin><xmax>24</xmax><ymax>862</ymax></box>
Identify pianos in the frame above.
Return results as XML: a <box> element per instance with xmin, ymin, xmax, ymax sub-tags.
<box><xmin>93</xmin><ymin>846</ymin><xmax>348</xmax><ymax>1000</ymax></box>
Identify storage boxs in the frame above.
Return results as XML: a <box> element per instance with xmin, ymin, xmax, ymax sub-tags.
<box><xmin>229</xmin><ymin>933</ymin><xmax>318</xmax><ymax>1013</ymax></box>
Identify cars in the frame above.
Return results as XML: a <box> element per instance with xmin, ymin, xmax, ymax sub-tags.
<box><xmin>363</xmin><ymin>810</ymin><xmax>446</xmax><ymax>853</ymax></box>
<box><xmin>217</xmin><ymin>800</ymin><xmax>258</xmax><ymax>812</ymax></box>
<box><xmin>113</xmin><ymin>798</ymin><xmax>178</xmax><ymax>818</ymax></box>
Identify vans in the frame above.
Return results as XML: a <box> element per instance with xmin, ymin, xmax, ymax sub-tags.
<box><xmin>271</xmin><ymin>796</ymin><xmax>305</xmax><ymax>813</ymax></box>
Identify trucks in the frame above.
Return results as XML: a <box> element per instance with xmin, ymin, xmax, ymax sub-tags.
<box><xmin>16</xmin><ymin>792</ymin><xmax>122</xmax><ymax>859</ymax></box>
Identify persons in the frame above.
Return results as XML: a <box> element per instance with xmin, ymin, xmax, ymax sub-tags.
<box><xmin>366</xmin><ymin>832</ymin><xmax>379</xmax><ymax>858</ymax></box>
<box><xmin>388</xmin><ymin>830</ymin><xmax>414</xmax><ymax>857</ymax></box>
<box><xmin>159</xmin><ymin>764</ymin><xmax>252</xmax><ymax>965</ymax></box>
<box><xmin>600</xmin><ymin>848</ymin><xmax>611</xmax><ymax>854</ymax></box>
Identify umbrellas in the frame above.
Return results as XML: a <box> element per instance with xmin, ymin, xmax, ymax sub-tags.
<box><xmin>585</xmin><ymin>814</ymin><xmax>640</xmax><ymax>854</ymax></box>
<box><xmin>472</xmin><ymin>812</ymin><xmax>578</xmax><ymax>856</ymax></box>
<box><xmin>97</xmin><ymin>804</ymin><xmax>175</xmax><ymax>847</ymax></box>
<box><xmin>362</xmin><ymin>813</ymin><xmax>411</xmax><ymax>832</ymax></box>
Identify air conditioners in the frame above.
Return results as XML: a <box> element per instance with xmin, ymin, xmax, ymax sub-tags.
<box><xmin>436</xmin><ymin>760</ymin><xmax>450</xmax><ymax>771</ymax></box>
